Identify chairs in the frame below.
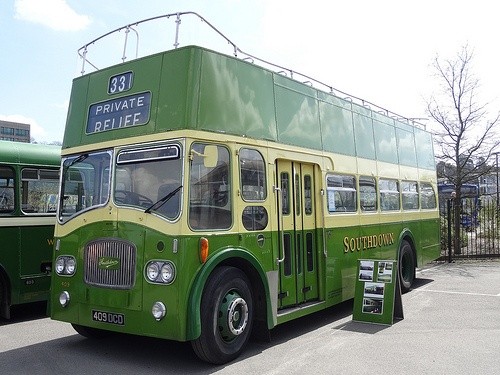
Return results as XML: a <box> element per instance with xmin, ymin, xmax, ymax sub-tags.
<box><xmin>153</xmin><ymin>184</ymin><xmax>179</xmax><ymax>218</ymax></box>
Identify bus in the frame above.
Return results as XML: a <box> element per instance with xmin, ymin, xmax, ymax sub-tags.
<box><xmin>0</xmin><ymin>139</ymin><xmax>62</xmax><ymax>320</ymax></box>
<box><xmin>439</xmin><ymin>183</ymin><xmax>484</xmax><ymax>232</ymax></box>
<box><xmin>48</xmin><ymin>11</ymin><xmax>442</xmax><ymax>365</ymax></box>
